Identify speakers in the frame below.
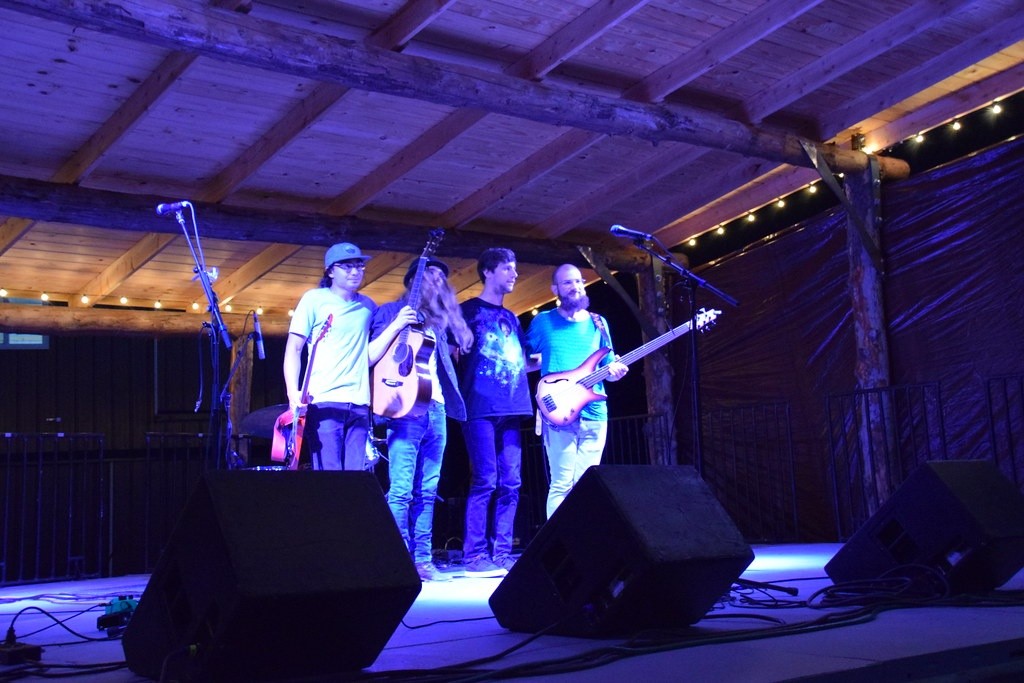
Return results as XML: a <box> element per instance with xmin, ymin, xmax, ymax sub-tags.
<box><xmin>824</xmin><ymin>460</ymin><xmax>1024</xmax><ymax>590</ymax></box>
<box><xmin>486</xmin><ymin>463</ymin><xmax>754</xmax><ymax>640</ymax></box>
<box><xmin>121</xmin><ymin>469</ymin><xmax>422</xmax><ymax>682</ymax></box>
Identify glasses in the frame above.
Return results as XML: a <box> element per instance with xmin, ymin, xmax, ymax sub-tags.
<box><xmin>333</xmin><ymin>262</ymin><xmax>366</xmax><ymax>270</ymax></box>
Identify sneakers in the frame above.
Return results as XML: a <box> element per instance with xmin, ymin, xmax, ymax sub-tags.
<box><xmin>464</xmin><ymin>553</ymin><xmax>516</xmax><ymax>578</ymax></box>
<box><xmin>415</xmin><ymin>561</ymin><xmax>453</xmax><ymax>583</ymax></box>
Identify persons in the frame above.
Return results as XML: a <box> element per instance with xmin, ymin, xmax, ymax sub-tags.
<box><xmin>524</xmin><ymin>263</ymin><xmax>630</xmax><ymax>523</ymax></box>
<box><xmin>369</xmin><ymin>256</ymin><xmax>474</xmax><ymax>582</ymax></box>
<box><xmin>283</xmin><ymin>241</ymin><xmax>382</xmax><ymax>471</ymax></box>
<box><xmin>457</xmin><ymin>248</ymin><xmax>535</xmax><ymax>579</ymax></box>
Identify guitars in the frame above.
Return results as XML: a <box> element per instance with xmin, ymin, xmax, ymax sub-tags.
<box><xmin>370</xmin><ymin>229</ymin><xmax>445</xmax><ymax>420</ymax></box>
<box><xmin>535</xmin><ymin>306</ymin><xmax>722</xmax><ymax>427</ymax></box>
<box><xmin>269</xmin><ymin>313</ymin><xmax>333</xmax><ymax>470</ymax></box>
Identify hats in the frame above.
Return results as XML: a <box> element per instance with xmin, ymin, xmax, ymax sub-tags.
<box><xmin>324</xmin><ymin>242</ymin><xmax>371</xmax><ymax>270</ymax></box>
<box><xmin>403</xmin><ymin>254</ymin><xmax>449</xmax><ymax>286</ymax></box>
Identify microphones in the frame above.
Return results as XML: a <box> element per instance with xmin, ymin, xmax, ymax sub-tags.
<box><xmin>254</xmin><ymin>313</ymin><xmax>266</xmax><ymax>359</ymax></box>
<box><xmin>610</xmin><ymin>224</ymin><xmax>652</xmax><ymax>241</ymax></box>
<box><xmin>156</xmin><ymin>201</ymin><xmax>188</xmax><ymax>216</ymax></box>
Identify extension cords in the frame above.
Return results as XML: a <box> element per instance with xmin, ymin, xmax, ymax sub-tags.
<box><xmin>0</xmin><ymin>639</ymin><xmax>43</xmax><ymax>664</ymax></box>
